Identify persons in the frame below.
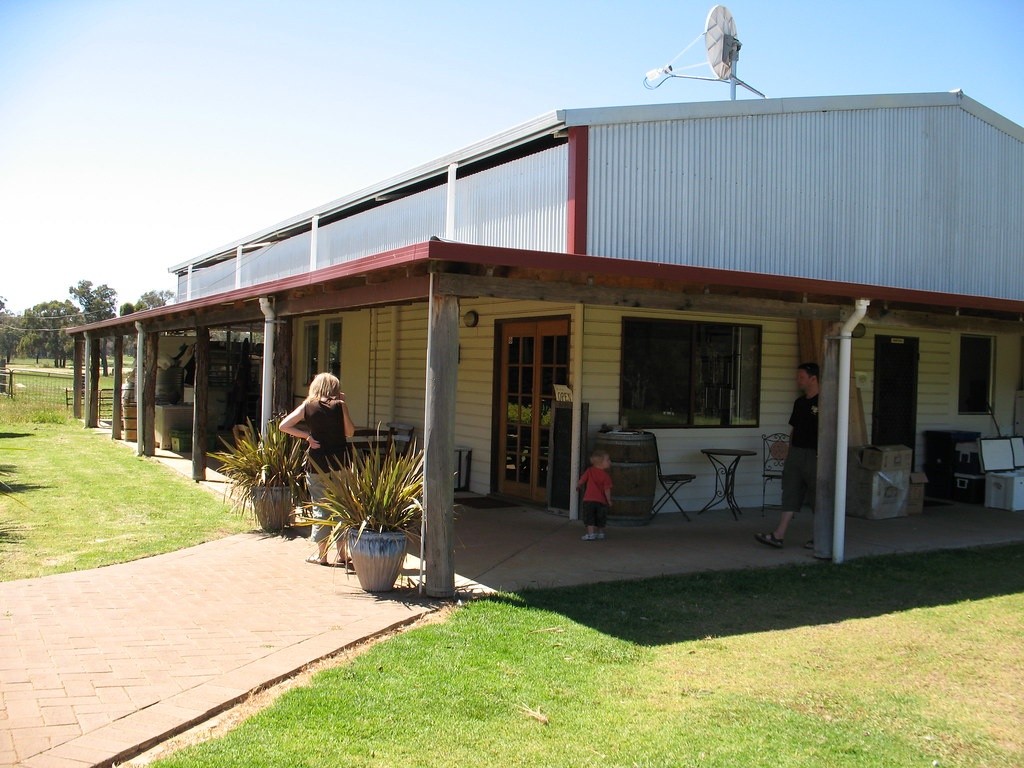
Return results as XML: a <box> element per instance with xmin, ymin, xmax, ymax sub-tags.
<box><xmin>576</xmin><ymin>449</ymin><xmax>612</xmax><ymax>541</ymax></box>
<box><xmin>278</xmin><ymin>372</ymin><xmax>355</xmax><ymax>564</ymax></box>
<box><xmin>753</xmin><ymin>362</ymin><xmax>820</xmax><ymax>549</ymax></box>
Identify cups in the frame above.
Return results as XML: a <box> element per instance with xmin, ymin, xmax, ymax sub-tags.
<box><xmin>620</xmin><ymin>417</ymin><xmax>628</xmax><ymax>432</ymax></box>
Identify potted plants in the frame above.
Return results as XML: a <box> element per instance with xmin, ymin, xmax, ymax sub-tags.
<box><xmin>204</xmin><ymin>412</ymin><xmax>310</xmax><ymax>531</ymax></box>
<box><xmin>284</xmin><ymin>421</ymin><xmax>465</xmax><ymax>593</ymax></box>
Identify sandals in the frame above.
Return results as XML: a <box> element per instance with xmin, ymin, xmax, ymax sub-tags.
<box><xmin>804</xmin><ymin>541</ymin><xmax>814</xmax><ymax>549</ymax></box>
<box><xmin>755</xmin><ymin>532</ymin><xmax>784</xmax><ymax>548</ymax></box>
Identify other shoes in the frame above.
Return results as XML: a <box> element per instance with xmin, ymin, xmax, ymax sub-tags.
<box><xmin>582</xmin><ymin>534</ymin><xmax>597</xmax><ymax>540</ymax></box>
<box><xmin>597</xmin><ymin>533</ymin><xmax>605</xmax><ymax>539</ymax></box>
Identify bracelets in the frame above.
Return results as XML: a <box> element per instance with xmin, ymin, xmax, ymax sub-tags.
<box><xmin>306</xmin><ymin>434</ymin><xmax>311</xmax><ymax>441</ymax></box>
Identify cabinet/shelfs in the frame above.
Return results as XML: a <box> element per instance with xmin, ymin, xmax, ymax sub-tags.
<box><xmin>153</xmin><ymin>404</ymin><xmax>193</xmax><ymax>450</ymax></box>
<box><xmin>209</xmin><ymin>351</ymin><xmax>261</xmax><ymax>426</ymax></box>
<box><xmin>925</xmin><ymin>430</ymin><xmax>980</xmax><ymax>472</ymax></box>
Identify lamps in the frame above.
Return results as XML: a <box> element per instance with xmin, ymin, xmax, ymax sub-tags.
<box><xmin>852</xmin><ymin>323</ymin><xmax>866</xmax><ymax>338</ymax></box>
<box><xmin>463</xmin><ymin>311</ymin><xmax>478</xmax><ymax>327</ymax></box>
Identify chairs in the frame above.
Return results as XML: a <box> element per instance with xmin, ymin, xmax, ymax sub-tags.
<box><xmin>650</xmin><ymin>435</ymin><xmax>696</xmax><ymax>522</ymax></box>
<box><xmin>364</xmin><ymin>422</ymin><xmax>415</xmax><ymax>473</ymax></box>
<box><xmin>761</xmin><ymin>433</ymin><xmax>795</xmax><ymax>519</ymax></box>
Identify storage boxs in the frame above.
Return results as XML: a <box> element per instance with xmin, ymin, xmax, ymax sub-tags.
<box><xmin>123</xmin><ymin>405</ymin><xmax>137</xmax><ymax>442</ymax></box>
<box><xmin>217</xmin><ymin>431</ymin><xmax>243</xmax><ymax>451</ymax></box>
<box><xmin>169</xmin><ymin>429</ymin><xmax>216</xmax><ymax>452</ymax></box>
<box><xmin>845</xmin><ymin>444</ymin><xmax>929</xmax><ymax>521</ymax></box>
<box><xmin>955</xmin><ymin>443</ymin><xmax>1024</xmax><ymax>512</ymax></box>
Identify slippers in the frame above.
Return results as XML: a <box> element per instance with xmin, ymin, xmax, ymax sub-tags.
<box><xmin>337</xmin><ymin>556</ymin><xmax>351</xmax><ymax>563</ymax></box>
<box><xmin>306</xmin><ymin>555</ymin><xmax>328</xmax><ymax>565</ymax></box>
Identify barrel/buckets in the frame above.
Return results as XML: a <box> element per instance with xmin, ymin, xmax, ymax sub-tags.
<box><xmin>155</xmin><ymin>367</ymin><xmax>183</xmax><ymax>405</ymax></box>
<box><xmin>597</xmin><ymin>432</ymin><xmax>658</xmax><ymax>526</ymax></box>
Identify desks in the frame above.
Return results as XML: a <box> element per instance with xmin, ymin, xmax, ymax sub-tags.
<box><xmin>454</xmin><ymin>446</ymin><xmax>473</xmax><ymax>491</ymax></box>
<box><xmin>344</xmin><ymin>426</ymin><xmax>397</xmax><ymax>467</ymax></box>
<box><xmin>697</xmin><ymin>449</ymin><xmax>757</xmax><ymax>520</ymax></box>
